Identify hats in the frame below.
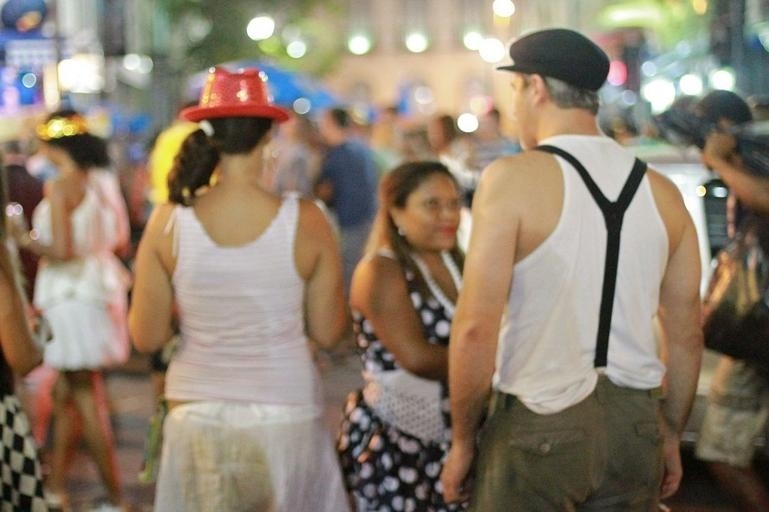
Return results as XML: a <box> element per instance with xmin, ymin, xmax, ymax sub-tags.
<box><xmin>179</xmin><ymin>66</ymin><xmax>290</xmax><ymax>124</ymax></box>
<box><xmin>496</xmin><ymin>27</ymin><xmax>610</xmax><ymax>92</ymax></box>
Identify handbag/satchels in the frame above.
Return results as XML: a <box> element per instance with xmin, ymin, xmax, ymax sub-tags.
<box><xmin>697</xmin><ymin>244</ymin><xmax>768</xmax><ymax>371</ymax></box>
